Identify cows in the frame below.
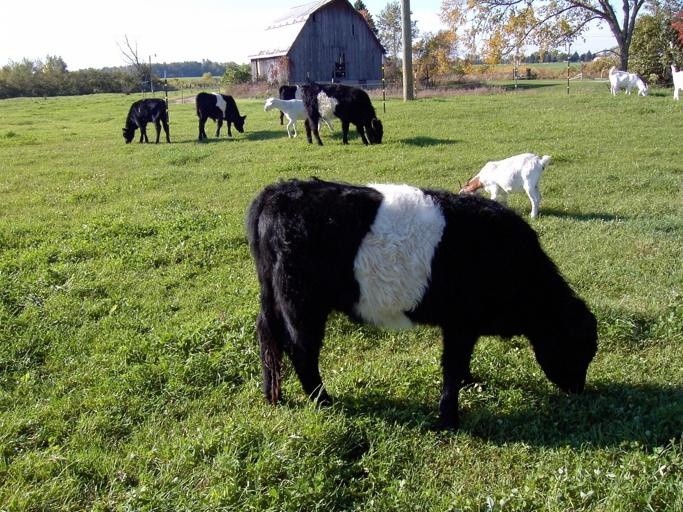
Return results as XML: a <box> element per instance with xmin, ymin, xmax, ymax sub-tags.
<box><xmin>122</xmin><ymin>98</ymin><xmax>169</xmax><ymax>144</ymax></box>
<box><xmin>279</xmin><ymin>85</ymin><xmax>302</xmax><ymax>125</ymax></box>
<box><xmin>245</xmin><ymin>175</ymin><xmax>598</xmax><ymax>430</ymax></box>
<box><xmin>300</xmin><ymin>83</ymin><xmax>383</xmax><ymax>146</ymax></box>
<box><xmin>196</xmin><ymin>93</ymin><xmax>246</xmax><ymax>141</ymax></box>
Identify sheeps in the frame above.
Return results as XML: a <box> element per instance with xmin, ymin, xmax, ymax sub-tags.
<box><xmin>671</xmin><ymin>64</ymin><xmax>683</xmax><ymax>100</ymax></box>
<box><xmin>458</xmin><ymin>153</ymin><xmax>553</xmax><ymax>219</ymax></box>
<box><xmin>264</xmin><ymin>97</ymin><xmax>334</xmax><ymax>139</ymax></box>
<box><xmin>609</xmin><ymin>66</ymin><xmax>649</xmax><ymax>97</ymax></box>
